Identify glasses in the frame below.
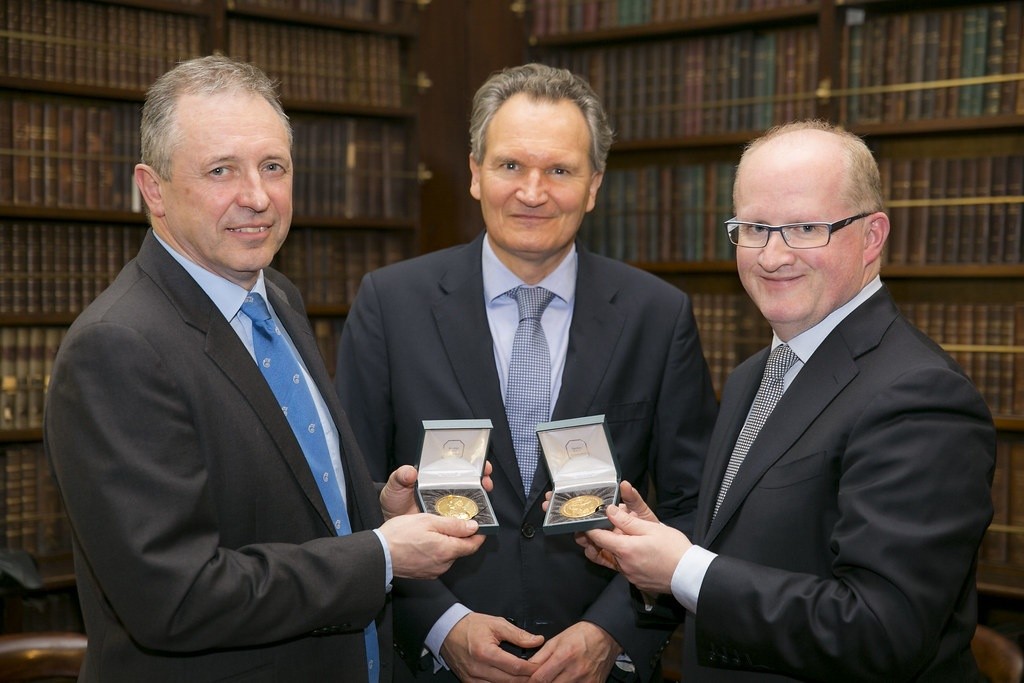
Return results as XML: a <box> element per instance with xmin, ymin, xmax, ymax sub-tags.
<box><xmin>723</xmin><ymin>212</ymin><xmax>870</xmax><ymax>249</ymax></box>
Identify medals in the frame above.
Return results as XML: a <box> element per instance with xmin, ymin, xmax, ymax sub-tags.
<box><xmin>562</xmin><ymin>495</ymin><xmax>605</xmax><ymax>517</ymax></box>
<box><xmin>435</xmin><ymin>495</ymin><xmax>480</xmax><ymax>521</ymax></box>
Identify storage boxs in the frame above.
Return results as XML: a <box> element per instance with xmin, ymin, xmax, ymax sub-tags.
<box><xmin>414</xmin><ymin>419</ymin><xmax>501</xmax><ymax>535</ymax></box>
<box><xmin>536</xmin><ymin>415</ymin><xmax>622</xmax><ymax>534</ymax></box>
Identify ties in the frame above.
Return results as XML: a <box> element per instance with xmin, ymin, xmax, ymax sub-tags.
<box><xmin>239</xmin><ymin>292</ymin><xmax>380</xmax><ymax>683</ymax></box>
<box><xmin>504</xmin><ymin>285</ymin><xmax>556</xmax><ymax>501</ymax></box>
<box><xmin>707</xmin><ymin>344</ymin><xmax>801</xmax><ymax>533</ymax></box>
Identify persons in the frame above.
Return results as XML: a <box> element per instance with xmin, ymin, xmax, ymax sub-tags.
<box><xmin>574</xmin><ymin>121</ymin><xmax>996</xmax><ymax>683</ymax></box>
<box><xmin>333</xmin><ymin>64</ymin><xmax>717</xmax><ymax>683</ymax></box>
<box><xmin>43</xmin><ymin>51</ymin><xmax>486</xmax><ymax>683</ymax></box>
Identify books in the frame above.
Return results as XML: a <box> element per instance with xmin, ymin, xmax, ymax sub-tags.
<box><xmin>0</xmin><ymin>0</ymin><xmax>1024</xmax><ymax>560</ymax></box>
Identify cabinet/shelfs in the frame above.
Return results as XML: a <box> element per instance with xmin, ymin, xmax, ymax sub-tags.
<box><xmin>0</xmin><ymin>0</ymin><xmax>1024</xmax><ymax>683</ymax></box>
